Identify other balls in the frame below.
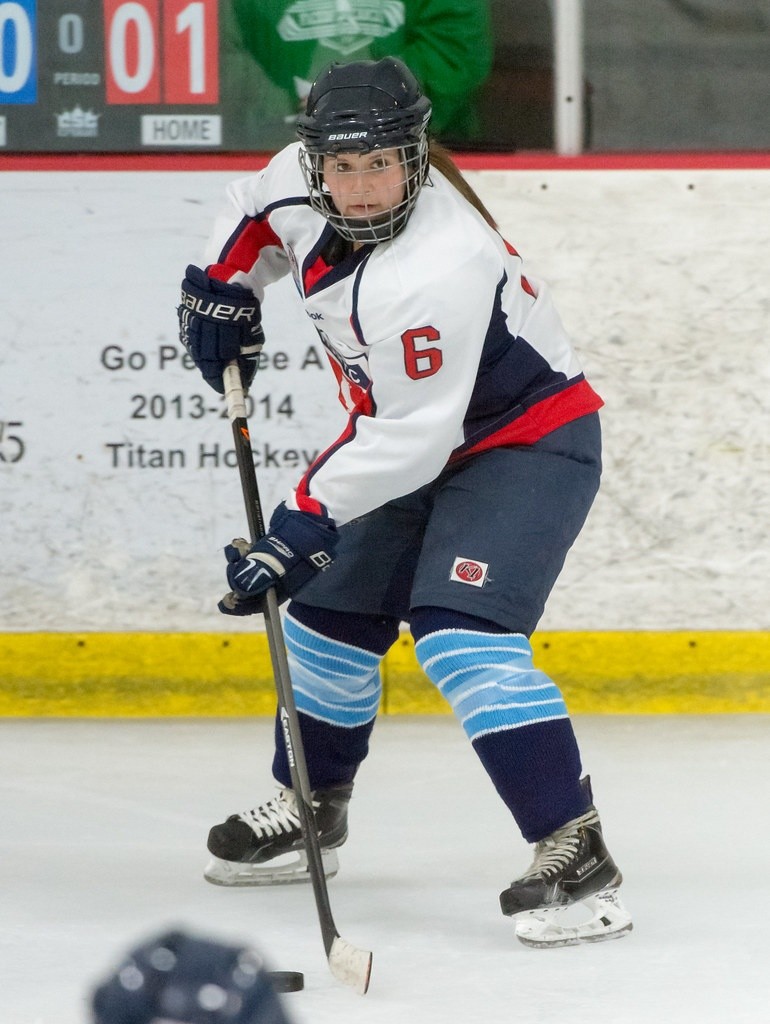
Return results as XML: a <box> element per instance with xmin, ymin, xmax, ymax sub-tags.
<box><xmin>270</xmin><ymin>971</ymin><xmax>305</xmax><ymax>992</ymax></box>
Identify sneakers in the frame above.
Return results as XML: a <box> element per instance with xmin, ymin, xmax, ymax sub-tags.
<box><xmin>498</xmin><ymin>776</ymin><xmax>634</xmax><ymax>948</ymax></box>
<box><xmin>205</xmin><ymin>781</ymin><xmax>353</xmax><ymax>887</ymax></box>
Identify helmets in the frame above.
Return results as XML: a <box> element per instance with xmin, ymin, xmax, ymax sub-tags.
<box><xmin>293</xmin><ymin>57</ymin><xmax>434</xmax><ymax>244</ymax></box>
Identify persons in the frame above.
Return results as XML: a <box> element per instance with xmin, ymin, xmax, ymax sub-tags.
<box><xmin>90</xmin><ymin>929</ymin><xmax>291</xmax><ymax>1024</ymax></box>
<box><xmin>218</xmin><ymin>0</ymin><xmax>496</xmax><ymax>151</ymax></box>
<box><xmin>178</xmin><ymin>59</ymin><xmax>638</xmax><ymax>946</ymax></box>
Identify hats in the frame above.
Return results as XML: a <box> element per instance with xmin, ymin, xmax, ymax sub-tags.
<box><xmin>94</xmin><ymin>933</ymin><xmax>287</xmax><ymax>1024</ymax></box>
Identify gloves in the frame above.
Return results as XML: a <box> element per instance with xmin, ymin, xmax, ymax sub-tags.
<box><xmin>217</xmin><ymin>501</ymin><xmax>342</xmax><ymax>615</ymax></box>
<box><xmin>176</xmin><ymin>265</ymin><xmax>266</xmax><ymax>397</ymax></box>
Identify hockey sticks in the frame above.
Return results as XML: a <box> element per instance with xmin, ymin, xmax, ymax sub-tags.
<box><xmin>224</xmin><ymin>346</ymin><xmax>374</xmax><ymax>992</ymax></box>
